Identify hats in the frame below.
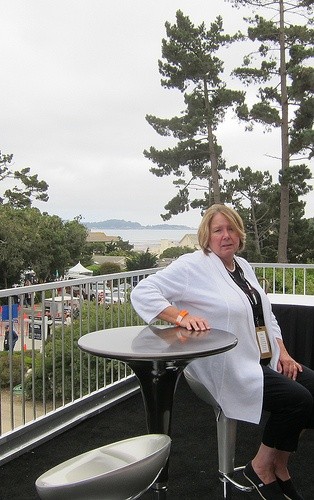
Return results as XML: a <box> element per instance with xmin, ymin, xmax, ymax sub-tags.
<box><xmin>5</xmin><ymin>325</ymin><xmax>9</xmax><ymax>327</ymax></box>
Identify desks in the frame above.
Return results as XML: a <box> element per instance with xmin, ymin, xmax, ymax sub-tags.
<box><xmin>78</xmin><ymin>324</ymin><xmax>237</xmax><ymax>500</ymax></box>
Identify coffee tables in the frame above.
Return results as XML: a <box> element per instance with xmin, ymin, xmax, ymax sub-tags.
<box><xmin>184</xmin><ymin>362</ymin><xmax>256</xmax><ymax>500</ymax></box>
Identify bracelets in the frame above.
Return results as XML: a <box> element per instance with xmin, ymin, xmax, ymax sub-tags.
<box><xmin>176</xmin><ymin>310</ymin><xmax>189</xmax><ymax>325</ymax></box>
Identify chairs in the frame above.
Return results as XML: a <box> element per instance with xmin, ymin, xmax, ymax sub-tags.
<box><xmin>35</xmin><ymin>434</ymin><xmax>172</xmax><ymax>500</ymax></box>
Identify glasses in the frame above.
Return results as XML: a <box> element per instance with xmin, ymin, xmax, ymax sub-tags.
<box><xmin>5</xmin><ymin>328</ymin><xmax>8</xmax><ymax>329</ymax></box>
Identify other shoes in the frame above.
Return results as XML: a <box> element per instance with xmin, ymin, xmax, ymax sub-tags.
<box><xmin>242</xmin><ymin>462</ymin><xmax>304</xmax><ymax>500</ymax></box>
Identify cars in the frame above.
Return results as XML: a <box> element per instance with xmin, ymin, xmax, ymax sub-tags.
<box><xmin>73</xmin><ymin>283</ymin><xmax>131</xmax><ymax>307</ymax></box>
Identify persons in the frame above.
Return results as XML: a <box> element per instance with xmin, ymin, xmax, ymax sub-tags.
<box><xmin>44</xmin><ymin>310</ymin><xmax>53</xmax><ymax>335</ymax></box>
<box><xmin>130</xmin><ymin>204</ymin><xmax>314</xmax><ymax>500</ymax></box>
<box><xmin>3</xmin><ymin>325</ymin><xmax>18</xmax><ymax>351</ymax></box>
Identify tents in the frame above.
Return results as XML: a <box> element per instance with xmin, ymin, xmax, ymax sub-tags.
<box><xmin>67</xmin><ymin>261</ymin><xmax>93</xmax><ymax>276</ymax></box>
